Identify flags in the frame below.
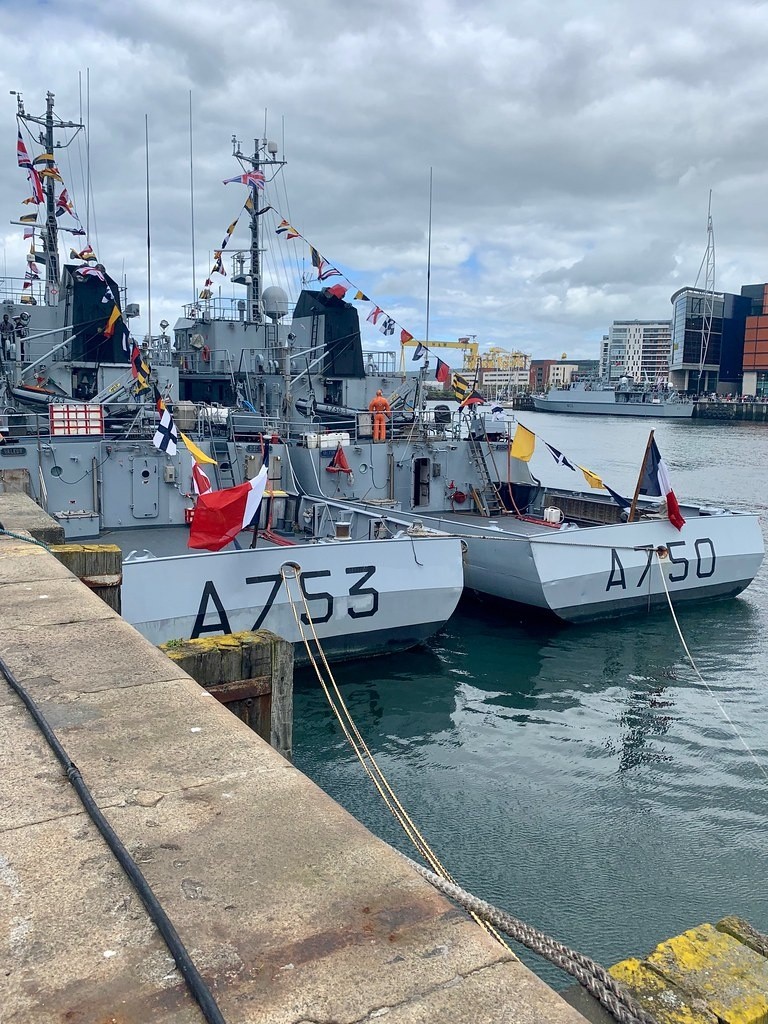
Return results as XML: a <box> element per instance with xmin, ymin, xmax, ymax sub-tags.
<box><xmin>19</xmin><ymin>170</ymin><xmax>44</xmax><ymax>291</ymax></box>
<box><xmin>222</xmin><ymin>170</ymin><xmax>264</xmax><ymax>190</ymax></box>
<box><xmin>638</xmin><ymin>437</ymin><xmax>686</xmax><ymax>532</ymax></box>
<box><xmin>32</xmin><ymin>153</ymin><xmax>217</xmax><ymax>495</ymax></box>
<box><xmin>189</xmin><ymin>193</ymin><xmax>253</xmax><ymax>317</ymax></box>
<box><xmin>187</xmin><ymin>453</ymin><xmax>269</xmax><ymax>552</ymax></box>
<box><xmin>254</xmin><ymin>205</ymin><xmax>631</xmax><ymax>513</ymax></box>
<box><xmin>17</xmin><ymin>127</ymin><xmax>45</xmax><ymax>203</ymax></box>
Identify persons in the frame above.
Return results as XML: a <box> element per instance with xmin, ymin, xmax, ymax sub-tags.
<box><xmin>12</xmin><ymin>316</ymin><xmax>24</xmax><ymax>361</ymax></box>
<box><xmin>707</xmin><ymin>392</ymin><xmax>767</xmax><ymax>402</ymax></box>
<box><xmin>369</xmin><ymin>389</ymin><xmax>391</xmax><ymax>444</ymax></box>
<box><xmin>0</xmin><ymin>314</ymin><xmax>15</xmax><ymax>361</ymax></box>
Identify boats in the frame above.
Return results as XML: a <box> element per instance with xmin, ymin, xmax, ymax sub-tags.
<box><xmin>0</xmin><ymin>65</ymin><xmax>467</xmax><ymax>667</ymax></box>
<box><xmin>530</xmin><ymin>376</ymin><xmax>696</xmax><ymax>419</ymax></box>
<box><xmin>140</xmin><ymin>90</ymin><xmax>766</xmax><ymax>625</ymax></box>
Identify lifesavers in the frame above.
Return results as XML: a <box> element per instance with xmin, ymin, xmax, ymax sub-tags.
<box><xmin>202</xmin><ymin>346</ymin><xmax>210</xmax><ymax>363</ymax></box>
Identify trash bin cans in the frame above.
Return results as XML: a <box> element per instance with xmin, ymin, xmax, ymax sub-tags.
<box><xmin>266</xmin><ymin>489</ymin><xmax>290</xmax><ymax>528</ymax></box>
<box><xmin>249</xmin><ymin>492</ymin><xmax>270</xmax><ymax>531</ymax></box>
